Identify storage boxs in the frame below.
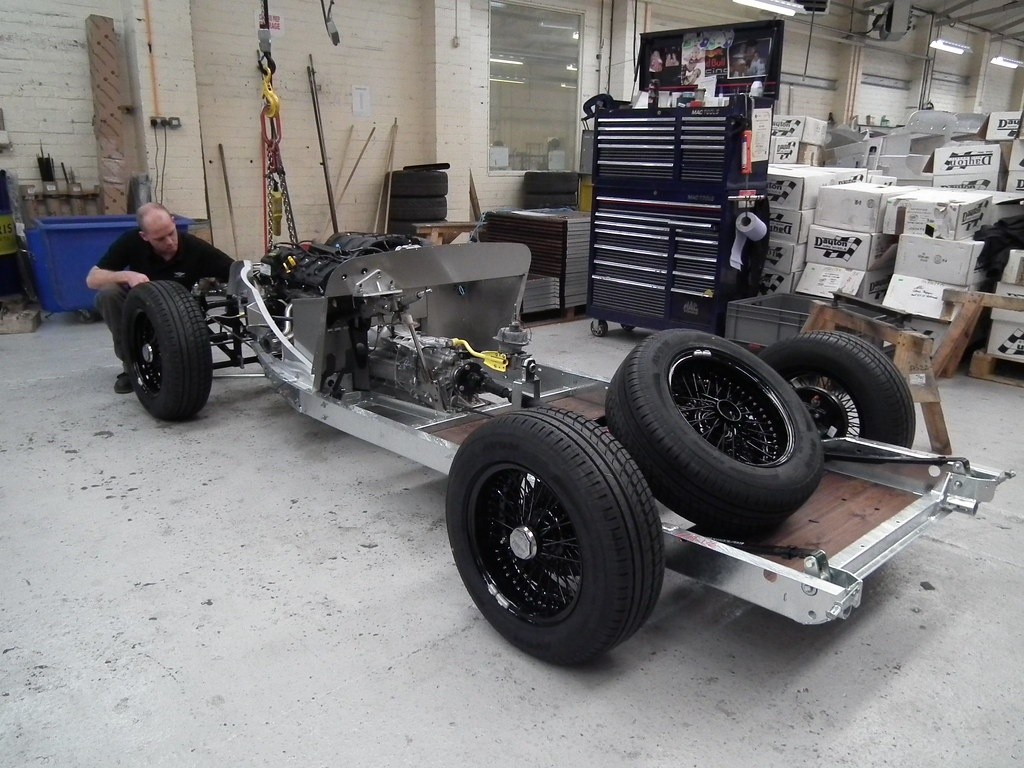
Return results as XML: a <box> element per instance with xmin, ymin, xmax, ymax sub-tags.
<box><xmin>23</xmin><ymin>212</ymin><xmax>192</xmax><ymax>311</ymax></box>
<box><xmin>756</xmin><ymin>111</ymin><xmax>1024</xmax><ymax>363</ymax></box>
<box><xmin>725</xmin><ymin>292</ymin><xmax>901</xmax><ymax>364</ymax></box>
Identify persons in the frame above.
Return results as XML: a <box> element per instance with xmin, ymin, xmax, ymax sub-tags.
<box><xmin>681</xmin><ymin>46</ymin><xmax>706</xmax><ymax>81</ymax></box>
<box><xmin>84</xmin><ymin>203</ymin><xmax>237</xmax><ymax>395</ymax></box>
<box><xmin>648</xmin><ymin>50</ymin><xmax>679</xmax><ymax>74</ymax></box>
<box><xmin>734</xmin><ymin>45</ymin><xmax>766</xmax><ymax>78</ymax></box>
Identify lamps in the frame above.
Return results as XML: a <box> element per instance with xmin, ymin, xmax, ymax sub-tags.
<box><xmin>541</xmin><ymin>20</ymin><xmax>577</xmax><ymax>31</ymax></box>
<box><xmin>490</xmin><ymin>74</ymin><xmax>527</xmax><ymax>85</ymax></box>
<box><xmin>733</xmin><ymin>1</ymin><xmax>806</xmax><ymax>17</ymax></box>
<box><xmin>930</xmin><ymin>0</ymin><xmax>973</xmax><ymax>55</ymax></box>
<box><xmin>561</xmin><ymin>82</ymin><xmax>576</xmax><ymax>89</ymax></box>
<box><xmin>990</xmin><ymin>11</ymin><xmax>1024</xmax><ymax>69</ymax></box>
<box><xmin>566</xmin><ymin>63</ymin><xmax>578</xmax><ymax>71</ymax></box>
<box><xmin>490</xmin><ymin>54</ymin><xmax>524</xmax><ymax>65</ymax></box>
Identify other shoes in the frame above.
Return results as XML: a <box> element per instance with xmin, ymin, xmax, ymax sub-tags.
<box><xmin>114</xmin><ymin>372</ymin><xmax>138</xmax><ymax>393</ymax></box>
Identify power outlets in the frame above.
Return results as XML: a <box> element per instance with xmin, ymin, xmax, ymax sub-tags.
<box><xmin>150</xmin><ymin>117</ymin><xmax>166</xmax><ymax>128</ymax></box>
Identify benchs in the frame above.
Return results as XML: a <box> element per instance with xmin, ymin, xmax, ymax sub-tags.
<box><xmin>930</xmin><ymin>289</ymin><xmax>1024</xmax><ymax>380</ymax></box>
<box><xmin>797</xmin><ymin>300</ymin><xmax>952</xmax><ymax>453</ymax></box>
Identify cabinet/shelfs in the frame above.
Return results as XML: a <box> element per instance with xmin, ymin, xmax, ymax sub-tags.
<box><xmin>489</xmin><ymin>146</ymin><xmax>509</xmax><ymax>170</ymax></box>
<box><xmin>547</xmin><ymin>150</ymin><xmax>565</xmax><ymax>170</ymax></box>
<box><xmin>585</xmin><ymin>94</ymin><xmax>774</xmax><ymax>338</ymax></box>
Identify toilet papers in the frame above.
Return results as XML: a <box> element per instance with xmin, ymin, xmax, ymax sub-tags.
<box><xmin>728</xmin><ymin>212</ymin><xmax>767</xmax><ymax>272</ymax></box>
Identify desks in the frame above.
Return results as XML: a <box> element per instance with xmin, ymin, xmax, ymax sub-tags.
<box><xmin>414</xmin><ymin>221</ymin><xmax>480</xmax><ymax>247</ymax></box>
<box><xmin>19</xmin><ymin>193</ymin><xmax>104</xmax><ymax>220</ymax></box>
